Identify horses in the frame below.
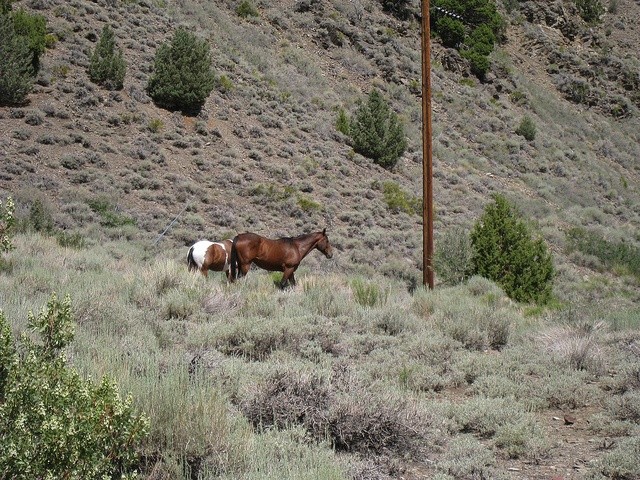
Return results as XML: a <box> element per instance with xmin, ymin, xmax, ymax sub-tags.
<box><xmin>185</xmin><ymin>238</ymin><xmax>238</xmax><ymax>288</ymax></box>
<box><xmin>231</xmin><ymin>227</ymin><xmax>334</xmax><ymax>291</ymax></box>
